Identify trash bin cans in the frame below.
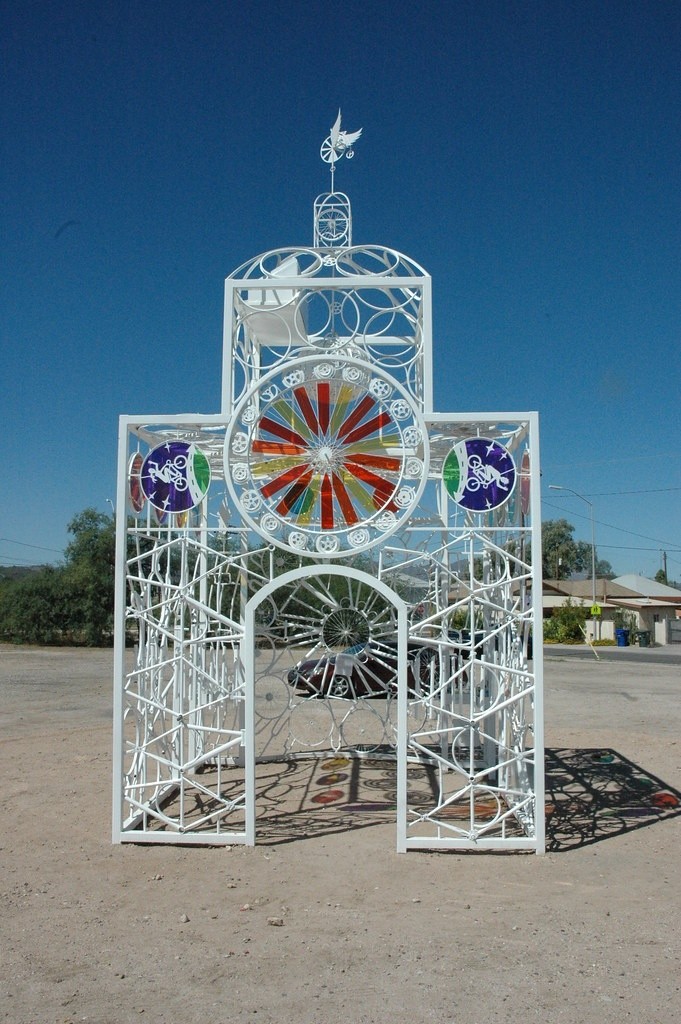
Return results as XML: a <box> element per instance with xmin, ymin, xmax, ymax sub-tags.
<box><xmin>615</xmin><ymin>629</ymin><xmax>630</xmax><ymax>647</ymax></box>
<box><xmin>636</xmin><ymin>630</ymin><xmax>652</xmax><ymax>647</ymax></box>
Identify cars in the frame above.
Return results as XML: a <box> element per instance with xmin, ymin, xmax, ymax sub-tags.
<box><xmin>286</xmin><ymin>640</ymin><xmax>469</xmax><ymax>700</ymax></box>
<box><xmin>447</xmin><ymin>623</ymin><xmax>532</xmax><ymax>661</ymax></box>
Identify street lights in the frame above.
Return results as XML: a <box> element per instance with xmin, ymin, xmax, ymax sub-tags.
<box><xmin>548</xmin><ymin>483</ymin><xmax>597</xmax><ymax>640</ymax></box>
